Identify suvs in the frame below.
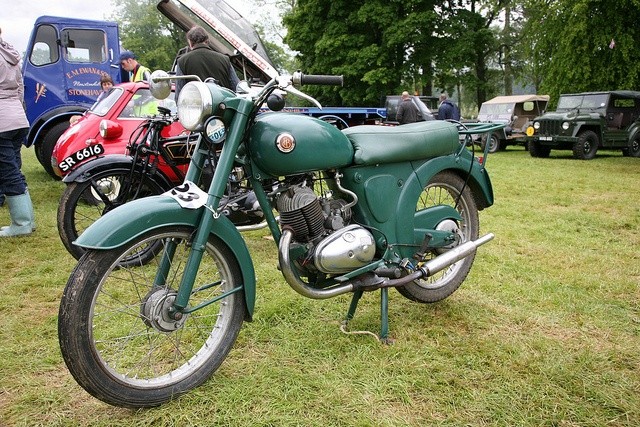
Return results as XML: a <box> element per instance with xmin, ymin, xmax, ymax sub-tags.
<box><xmin>382</xmin><ymin>94</ymin><xmax>439</xmax><ymax>126</ymax></box>
<box><xmin>527</xmin><ymin>89</ymin><xmax>640</xmax><ymax>161</ymax></box>
<box><xmin>475</xmin><ymin>93</ymin><xmax>551</xmax><ymax>154</ymax></box>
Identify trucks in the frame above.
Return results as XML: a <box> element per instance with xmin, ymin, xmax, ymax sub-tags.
<box><xmin>20</xmin><ymin>14</ymin><xmax>390</xmax><ymax>180</ymax></box>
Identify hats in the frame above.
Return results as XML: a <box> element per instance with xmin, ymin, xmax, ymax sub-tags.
<box><xmin>114</xmin><ymin>51</ymin><xmax>135</xmax><ymax>64</ymax></box>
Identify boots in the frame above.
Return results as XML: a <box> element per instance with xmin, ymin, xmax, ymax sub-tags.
<box><xmin>0</xmin><ymin>194</ymin><xmax>32</xmax><ymax>238</ymax></box>
<box><xmin>1</xmin><ymin>185</ymin><xmax>36</xmax><ymax>232</ymax></box>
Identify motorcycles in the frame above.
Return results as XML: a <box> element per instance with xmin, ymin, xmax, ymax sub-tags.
<box><xmin>55</xmin><ymin>107</ymin><xmax>315</xmax><ymax>271</ymax></box>
<box><xmin>55</xmin><ymin>70</ymin><xmax>510</xmax><ymax>408</ymax></box>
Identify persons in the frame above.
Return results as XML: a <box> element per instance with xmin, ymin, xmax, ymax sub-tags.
<box><xmin>397</xmin><ymin>91</ymin><xmax>421</xmax><ymax>123</ymax></box>
<box><xmin>174</xmin><ymin>26</ymin><xmax>240</xmax><ymax>105</ymax></box>
<box><xmin>435</xmin><ymin>94</ymin><xmax>459</xmax><ymax>120</ymax></box>
<box><xmin>0</xmin><ymin>28</ymin><xmax>35</xmax><ymax>237</ymax></box>
<box><xmin>114</xmin><ymin>51</ymin><xmax>159</xmax><ymax>116</ymax></box>
<box><xmin>97</xmin><ymin>74</ymin><xmax>113</xmax><ymax>101</ymax></box>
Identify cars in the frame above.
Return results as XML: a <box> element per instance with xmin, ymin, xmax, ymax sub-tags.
<box><xmin>52</xmin><ymin>79</ymin><xmax>202</xmax><ymax>204</ymax></box>
